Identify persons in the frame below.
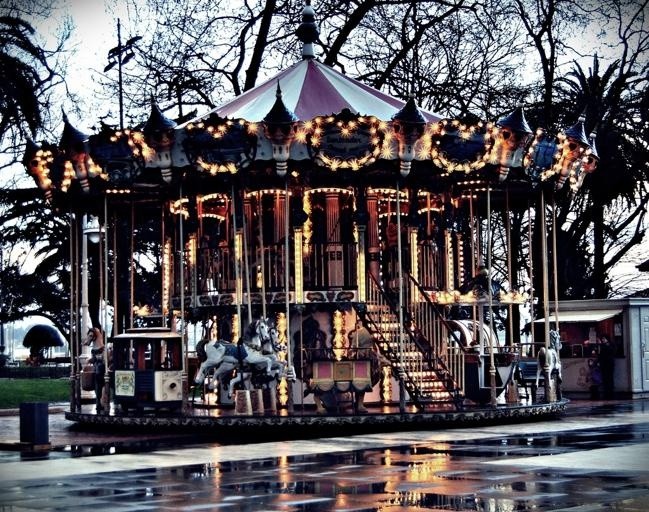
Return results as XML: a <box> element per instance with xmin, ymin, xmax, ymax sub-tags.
<box><xmin>587</xmin><ymin>358</ymin><xmax>602</xmax><ymax>398</ymax></box>
<box><xmin>597</xmin><ymin>334</ymin><xmax>615</xmax><ymax>400</ymax></box>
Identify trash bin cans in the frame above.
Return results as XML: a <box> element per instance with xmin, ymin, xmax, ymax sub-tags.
<box><xmin>19</xmin><ymin>402</ymin><xmax>48</xmax><ymax>461</ymax></box>
